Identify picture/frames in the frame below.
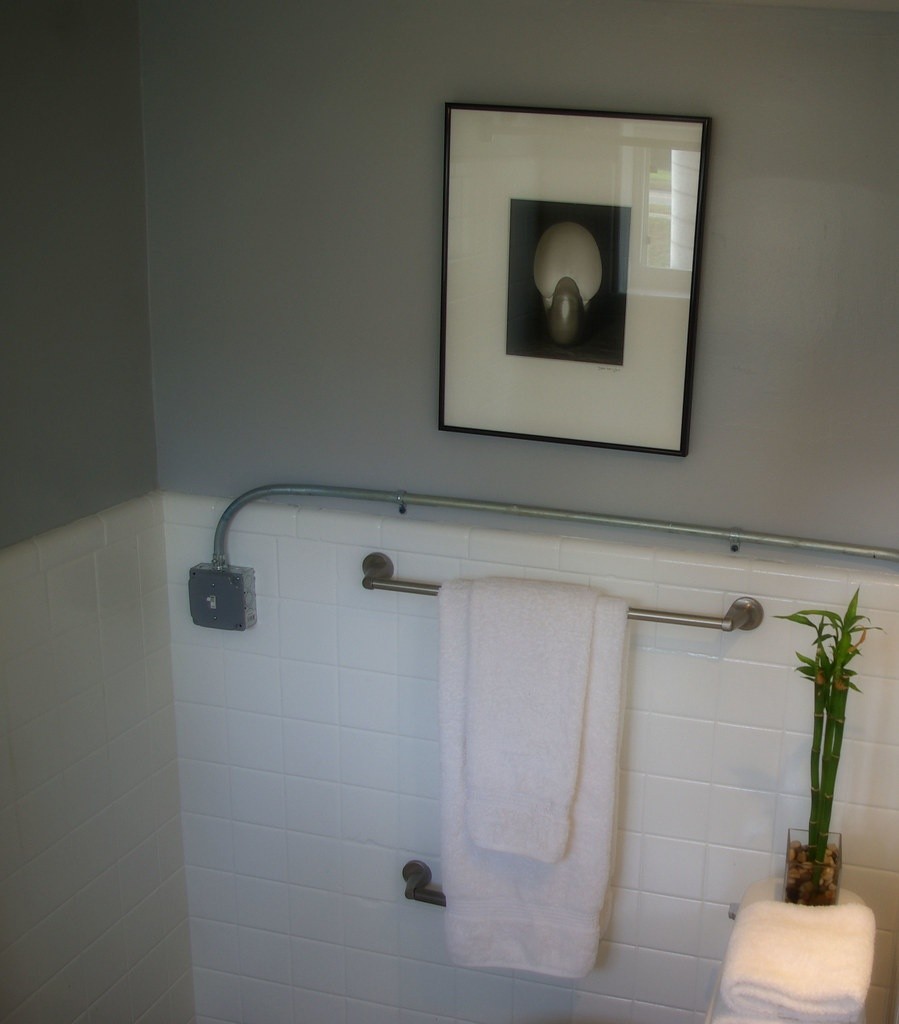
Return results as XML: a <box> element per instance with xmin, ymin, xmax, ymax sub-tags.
<box><xmin>439</xmin><ymin>102</ymin><xmax>712</xmax><ymax>456</ymax></box>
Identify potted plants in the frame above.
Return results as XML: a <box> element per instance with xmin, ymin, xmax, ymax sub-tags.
<box><xmin>772</xmin><ymin>583</ymin><xmax>888</xmax><ymax>906</ymax></box>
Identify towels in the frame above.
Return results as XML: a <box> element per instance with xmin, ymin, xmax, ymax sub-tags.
<box><xmin>704</xmin><ymin>876</ymin><xmax>877</xmax><ymax>1024</ymax></box>
<box><xmin>437</xmin><ymin>575</ymin><xmax>630</xmax><ymax>980</ymax></box>
